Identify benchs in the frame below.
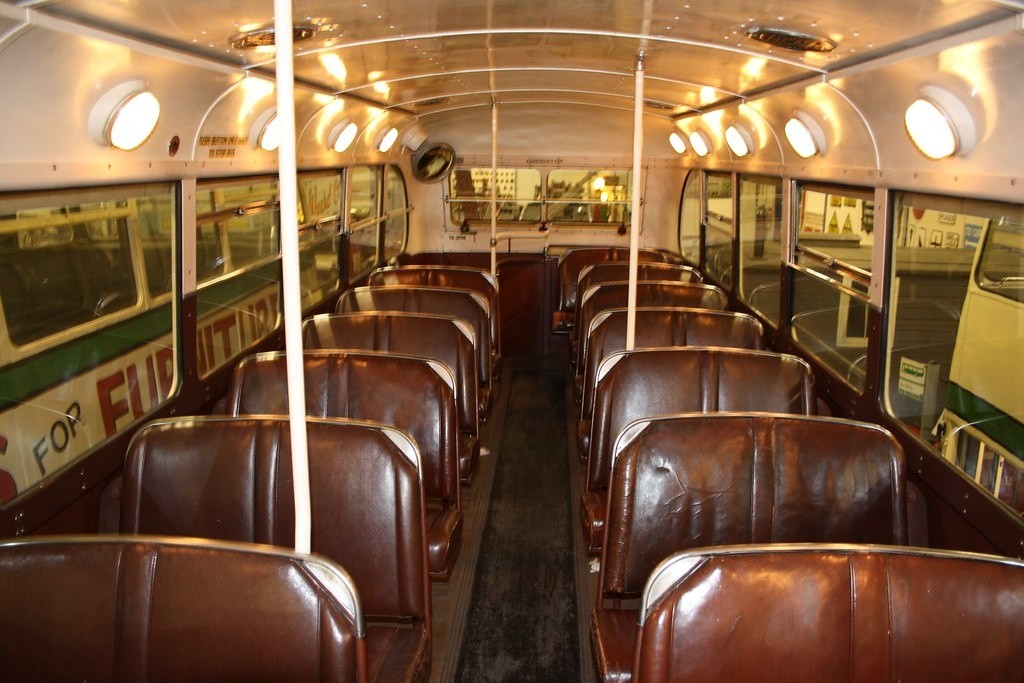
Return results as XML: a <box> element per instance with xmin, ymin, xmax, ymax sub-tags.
<box><xmin>1</xmin><ymin>245</ymin><xmax>1024</xmax><ymax>683</ymax></box>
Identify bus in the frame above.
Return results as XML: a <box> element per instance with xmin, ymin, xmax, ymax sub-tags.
<box><xmin>0</xmin><ymin>165</ymin><xmax>402</xmax><ymax>504</ymax></box>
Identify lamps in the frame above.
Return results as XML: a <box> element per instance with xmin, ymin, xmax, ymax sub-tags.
<box><xmin>667</xmin><ymin>106</ymin><xmax>829</xmax><ymax>157</ymax></box>
<box><xmin>903</xmin><ymin>84</ymin><xmax>978</xmax><ymax>161</ymax></box>
<box><xmin>246</xmin><ymin>101</ymin><xmax>429</xmax><ymax>156</ymax></box>
<box><xmin>85</xmin><ymin>77</ymin><xmax>165</xmax><ymax>154</ymax></box>
